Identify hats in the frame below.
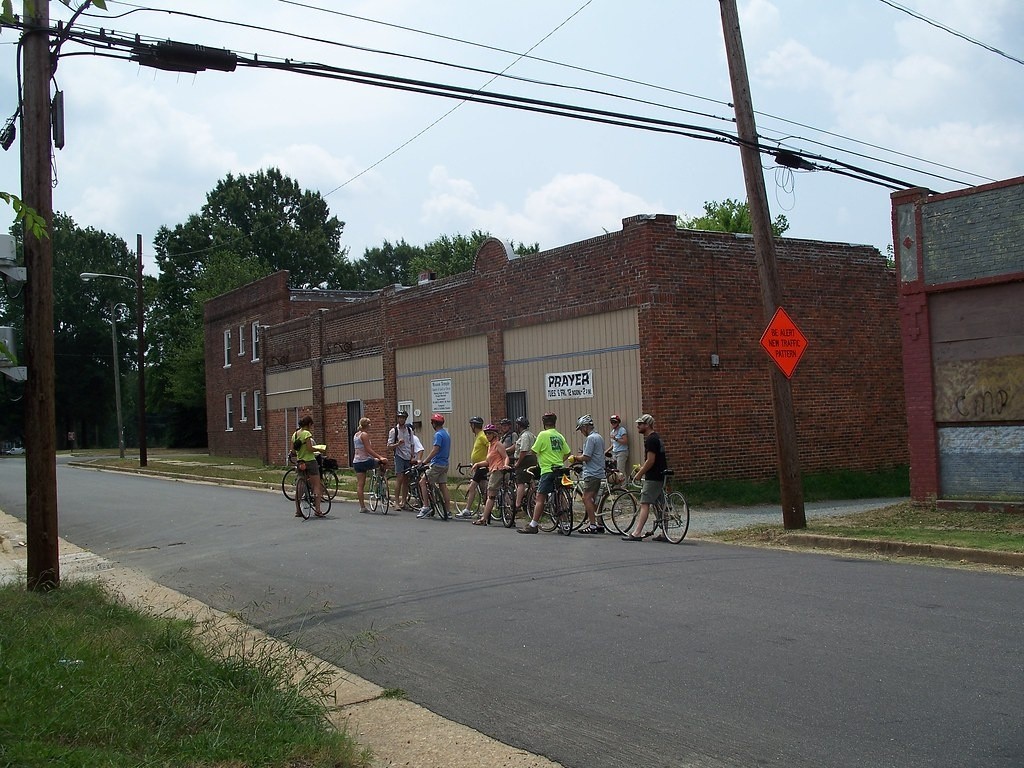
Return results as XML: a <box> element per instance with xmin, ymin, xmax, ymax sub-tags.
<box><xmin>635</xmin><ymin>414</ymin><xmax>653</xmax><ymax>426</ymax></box>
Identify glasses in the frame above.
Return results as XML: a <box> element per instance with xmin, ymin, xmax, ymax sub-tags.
<box><xmin>611</xmin><ymin>421</ymin><xmax>617</xmax><ymax>424</ymax></box>
<box><xmin>485</xmin><ymin>431</ymin><xmax>493</xmax><ymax>435</ymax></box>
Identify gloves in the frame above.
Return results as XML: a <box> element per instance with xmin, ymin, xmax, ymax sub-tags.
<box><xmin>568</xmin><ymin>456</ymin><xmax>576</xmax><ymax>463</ymax></box>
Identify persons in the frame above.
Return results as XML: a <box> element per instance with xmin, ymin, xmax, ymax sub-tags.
<box><xmin>622</xmin><ymin>414</ymin><xmax>670</xmax><ymax>542</ymax></box>
<box><xmin>455</xmin><ymin>411</ymin><xmax>572</xmax><ymax>534</ymax></box>
<box><xmin>416</xmin><ymin>414</ymin><xmax>453</xmax><ymax>519</ymax></box>
<box><xmin>353</xmin><ymin>418</ymin><xmax>389</xmax><ymax>513</ymax></box>
<box><xmin>387</xmin><ymin>410</ymin><xmax>425</xmax><ymax>511</ymax></box>
<box><xmin>291</xmin><ymin>416</ymin><xmax>327</xmax><ymax>517</ymax></box>
<box><xmin>568</xmin><ymin>414</ymin><xmax>605</xmax><ymax>534</ymax></box>
<box><xmin>605</xmin><ymin>415</ymin><xmax>630</xmax><ymax>489</ymax></box>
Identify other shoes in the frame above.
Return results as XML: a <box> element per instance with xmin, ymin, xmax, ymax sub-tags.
<box><xmin>621</xmin><ymin>533</ymin><xmax>642</xmax><ymax>541</ymax></box>
<box><xmin>359</xmin><ymin>507</ymin><xmax>370</xmax><ymax>512</ymax></box>
<box><xmin>394</xmin><ymin>505</ymin><xmax>401</xmax><ymax>511</ymax></box>
<box><xmin>652</xmin><ymin>535</ymin><xmax>668</xmax><ymax>542</ymax></box>
<box><xmin>402</xmin><ymin>504</ymin><xmax>413</xmax><ymax>511</ymax></box>
<box><xmin>473</xmin><ymin>517</ymin><xmax>490</xmax><ymax>526</ymax></box>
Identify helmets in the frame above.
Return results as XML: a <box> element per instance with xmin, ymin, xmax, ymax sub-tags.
<box><xmin>483</xmin><ymin>424</ymin><xmax>498</xmax><ymax>434</ymax></box>
<box><xmin>516</xmin><ymin>416</ymin><xmax>529</xmax><ymax>428</ymax></box>
<box><xmin>500</xmin><ymin>419</ymin><xmax>510</xmax><ymax>424</ymax></box>
<box><xmin>431</xmin><ymin>414</ymin><xmax>444</xmax><ymax>422</ymax></box>
<box><xmin>397</xmin><ymin>410</ymin><xmax>408</xmax><ymax>417</ymax></box>
<box><xmin>610</xmin><ymin>415</ymin><xmax>621</xmax><ymax>423</ymax></box>
<box><xmin>470</xmin><ymin>416</ymin><xmax>482</xmax><ymax>424</ymax></box>
<box><xmin>576</xmin><ymin>415</ymin><xmax>593</xmax><ymax>431</ymax></box>
<box><xmin>542</xmin><ymin>411</ymin><xmax>557</xmax><ymax>422</ymax></box>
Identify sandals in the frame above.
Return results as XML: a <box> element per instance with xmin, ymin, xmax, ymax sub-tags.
<box><xmin>314</xmin><ymin>511</ymin><xmax>326</xmax><ymax>517</ymax></box>
<box><xmin>598</xmin><ymin>527</ymin><xmax>605</xmax><ymax>533</ymax></box>
<box><xmin>295</xmin><ymin>512</ymin><xmax>302</xmax><ymax>517</ymax></box>
<box><xmin>578</xmin><ymin>527</ymin><xmax>599</xmax><ymax>534</ymax></box>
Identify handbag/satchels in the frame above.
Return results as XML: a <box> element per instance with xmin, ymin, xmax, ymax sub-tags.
<box><xmin>293</xmin><ymin>431</ymin><xmax>306</xmax><ymax>450</ymax></box>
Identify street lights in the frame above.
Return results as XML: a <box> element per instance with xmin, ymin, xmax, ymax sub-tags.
<box><xmin>111</xmin><ymin>301</ymin><xmax>127</xmax><ymax>457</ymax></box>
<box><xmin>79</xmin><ymin>271</ymin><xmax>146</xmax><ymax>469</ymax></box>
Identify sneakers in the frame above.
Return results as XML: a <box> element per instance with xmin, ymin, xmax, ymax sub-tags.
<box><xmin>517</xmin><ymin>525</ymin><xmax>539</xmax><ymax>534</ymax></box>
<box><xmin>456</xmin><ymin>511</ymin><xmax>472</xmax><ymax>519</ymax></box>
<box><xmin>447</xmin><ymin>511</ymin><xmax>452</xmax><ymax>518</ymax></box>
<box><xmin>416</xmin><ymin>508</ymin><xmax>430</xmax><ymax>518</ymax></box>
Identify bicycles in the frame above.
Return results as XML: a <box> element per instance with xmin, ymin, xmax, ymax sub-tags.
<box><xmin>280</xmin><ymin>449</ymin><xmax>690</xmax><ymax>545</ymax></box>
